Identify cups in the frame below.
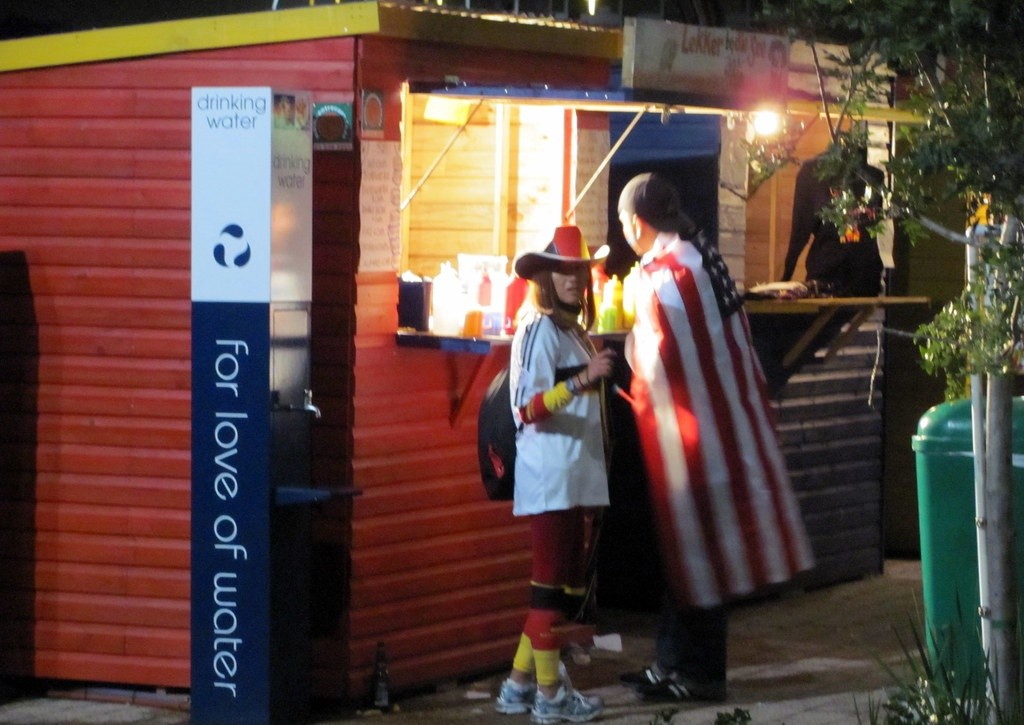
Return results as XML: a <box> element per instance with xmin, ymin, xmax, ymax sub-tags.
<box><xmin>399</xmin><ymin>280</ymin><xmax>431</xmax><ymax>332</ymax></box>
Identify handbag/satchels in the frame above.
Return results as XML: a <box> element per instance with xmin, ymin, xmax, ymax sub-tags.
<box><xmin>432</xmin><ymin>260</ymin><xmax>459</xmax><ymax>333</ymax></box>
<box><xmin>458</xmin><ymin>255</ymin><xmax>509</xmax><ymax>335</ymax></box>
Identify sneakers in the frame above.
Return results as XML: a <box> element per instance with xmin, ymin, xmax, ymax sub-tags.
<box><xmin>531</xmin><ymin>681</ymin><xmax>604</xmax><ymax>725</ymax></box>
<box><xmin>620</xmin><ymin>661</ymin><xmax>669</xmax><ymax>685</ymax></box>
<box><xmin>636</xmin><ymin>671</ymin><xmax>727</xmax><ymax>702</ymax></box>
<box><xmin>495</xmin><ymin>677</ymin><xmax>536</xmax><ymax>715</ymax></box>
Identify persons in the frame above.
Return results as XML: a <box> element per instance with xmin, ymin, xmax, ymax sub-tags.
<box><xmin>493</xmin><ymin>236</ymin><xmax>619</xmax><ymax>724</ymax></box>
<box><xmin>606</xmin><ymin>173</ymin><xmax>734</xmax><ymax>705</ymax></box>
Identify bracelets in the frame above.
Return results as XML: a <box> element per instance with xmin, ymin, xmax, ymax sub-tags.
<box><xmin>586</xmin><ymin>365</ymin><xmax>592</xmax><ymax>385</ymax></box>
<box><xmin>576</xmin><ymin>372</ymin><xmax>585</xmax><ymax>388</ymax></box>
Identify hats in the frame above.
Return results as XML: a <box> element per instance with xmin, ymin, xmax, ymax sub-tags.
<box><xmin>514</xmin><ymin>226</ymin><xmax>611</xmax><ymax>280</ymax></box>
<box><xmin>620</xmin><ymin>173</ymin><xmax>694</xmax><ymax>232</ymax></box>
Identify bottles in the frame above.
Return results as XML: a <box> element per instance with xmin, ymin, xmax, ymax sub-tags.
<box><xmin>429</xmin><ymin>253</ymin><xmax>641</xmax><ymax>341</ymax></box>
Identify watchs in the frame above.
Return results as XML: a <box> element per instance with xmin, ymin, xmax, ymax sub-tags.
<box><xmin>564</xmin><ymin>378</ymin><xmax>579</xmax><ymax>396</ymax></box>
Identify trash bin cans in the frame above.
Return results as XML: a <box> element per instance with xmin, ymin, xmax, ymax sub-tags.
<box><xmin>911</xmin><ymin>395</ymin><xmax>1024</xmax><ymax>703</ymax></box>
